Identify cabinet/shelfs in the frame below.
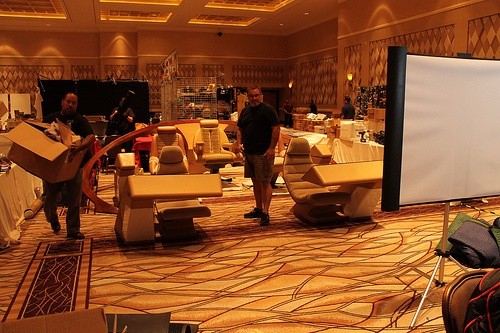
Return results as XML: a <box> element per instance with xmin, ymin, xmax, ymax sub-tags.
<box><xmin>176</xmin><ymin>91</ymin><xmax>217</xmax><ymax>118</ymax></box>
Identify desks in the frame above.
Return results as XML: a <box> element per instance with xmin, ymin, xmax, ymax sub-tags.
<box><xmin>0</xmin><ymin>133</ymin><xmax>43</xmax><ymax>249</ymax></box>
<box><xmin>327</xmin><ymin>138</ymin><xmax>383</xmax><ymax>166</ymax></box>
<box><xmin>132</xmin><ymin>137</ymin><xmax>152</xmax><ymax>168</ymax></box>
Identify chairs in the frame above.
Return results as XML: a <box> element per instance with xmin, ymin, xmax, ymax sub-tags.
<box><xmin>283</xmin><ymin>137</ymin><xmax>351</xmax><ymax>222</ymax></box>
<box><xmin>100</xmin><ymin>121</ymin><xmax>235</xmax><ymax>231</ymax></box>
<box><xmin>442</xmin><ymin>269</ymin><xmax>500</xmax><ymax>333</ymax></box>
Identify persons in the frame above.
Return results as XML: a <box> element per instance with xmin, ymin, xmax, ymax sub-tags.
<box><xmin>309</xmin><ymin>99</ymin><xmax>317</xmax><ymax>113</ymax></box>
<box><xmin>340</xmin><ymin>96</ymin><xmax>354</xmax><ymax>120</ymax></box>
<box><xmin>236</xmin><ymin>83</ymin><xmax>281</xmax><ymax>226</ymax></box>
<box><xmin>42</xmin><ymin>92</ymin><xmax>135</xmax><ymax>238</ymax></box>
<box><xmin>283</xmin><ymin>99</ymin><xmax>294</xmax><ymax>128</ymax></box>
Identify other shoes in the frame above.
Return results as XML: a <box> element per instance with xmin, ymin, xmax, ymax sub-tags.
<box><xmin>51</xmin><ymin>223</ymin><xmax>62</xmax><ymax>232</ymax></box>
<box><xmin>244</xmin><ymin>207</ymin><xmax>262</xmax><ymax>218</ymax></box>
<box><xmin>260</xmin><ymin>212</ymin><xmax>269</xmax><ymax>225</ymax></box>
<box><xmin>67</xmin><ymin>232</ymin><xmax>86</xmax><ymax>240</ymax></box>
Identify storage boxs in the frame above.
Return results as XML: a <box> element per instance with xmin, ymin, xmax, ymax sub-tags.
<box><xmin>367</xmin><ymin>107</ymin><xmax>386</xmax><ymax>122</ymax></box>
<box><xmin>4</xmin><ymin>120</ymin><xmax>89</xmax><ymax>182</ymax></box>
